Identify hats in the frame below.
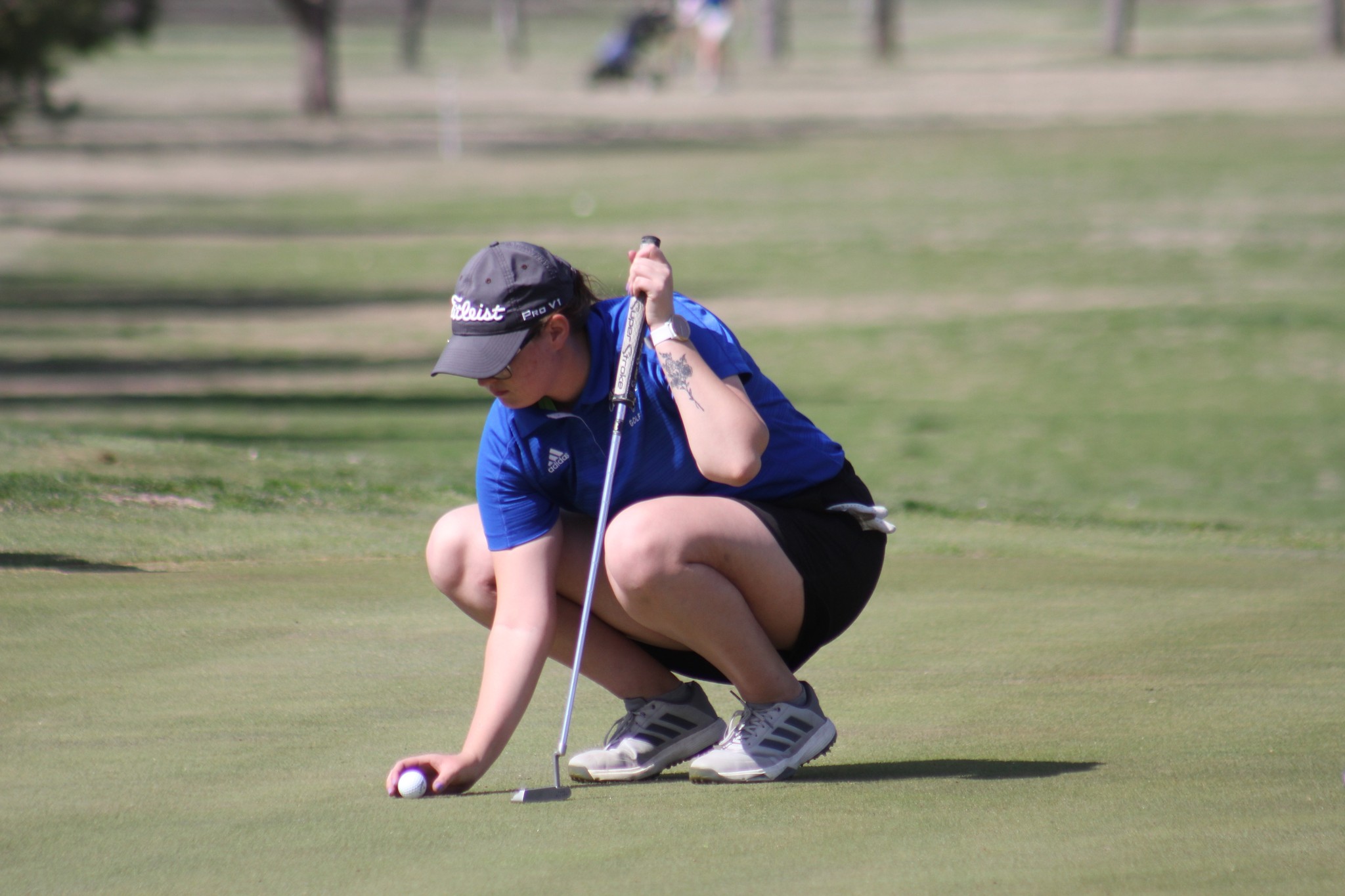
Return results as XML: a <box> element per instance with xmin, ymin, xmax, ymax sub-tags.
<box><xmin>430</xmin><ymin>239</ymin><xmax>572</xmax><ymax>378</ymax></box>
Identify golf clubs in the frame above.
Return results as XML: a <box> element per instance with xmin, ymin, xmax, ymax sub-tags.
<box><xmin>506</xmin><ymin>231</ymin><xmax>666</xmax><ymax>804</ymax></box>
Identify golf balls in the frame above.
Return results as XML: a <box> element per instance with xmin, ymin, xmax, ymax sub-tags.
<box><xmin>397</xmin><ymin>770</ymin><xmax>427</xmax><ymax>799</ymax></box>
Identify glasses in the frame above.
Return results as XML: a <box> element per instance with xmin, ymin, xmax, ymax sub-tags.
<box><xmin>490</xmin><ymin>326</ymin><xmax>539</xmax><ymax>377</ymax></box>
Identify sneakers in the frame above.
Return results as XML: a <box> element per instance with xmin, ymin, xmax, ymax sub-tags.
<box><xmin>689</xmin><ymin>679</ymin><xmax>837</xmax><ymax>780</ymax></box>
<box><xmin>570</xmin><ymin>681</ymin><xmax>728</xmax><ymax>783</ymax></box>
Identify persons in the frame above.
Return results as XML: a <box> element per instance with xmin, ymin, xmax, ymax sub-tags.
<box><xmin>385</xmin><ymin>239</ymin><xmax>897</xmax><ymax>806</ymax></box>
<box><xmin>678</xmin><ymin>0</ymin><xmax>732</xmax><ymax>80</ymax></box>
